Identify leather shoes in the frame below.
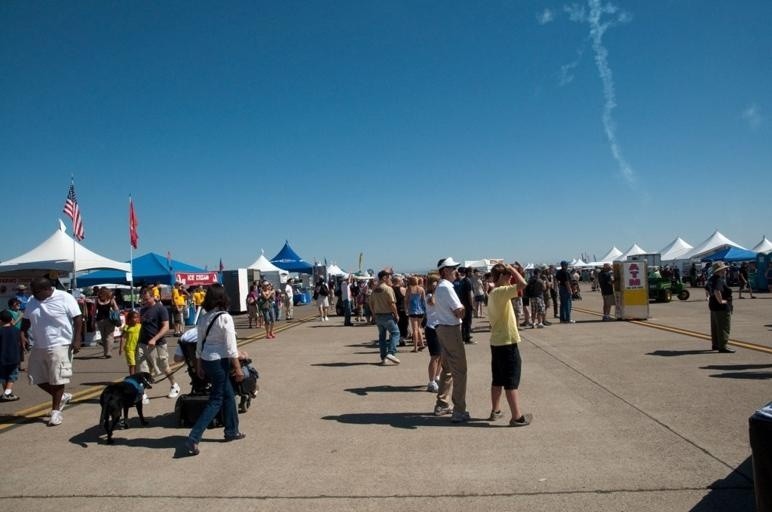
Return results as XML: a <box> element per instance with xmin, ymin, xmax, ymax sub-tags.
<box><xmin>712</xmin><ymin>345</ymin><xmax>737</xmax><ymax>353</ymax></box>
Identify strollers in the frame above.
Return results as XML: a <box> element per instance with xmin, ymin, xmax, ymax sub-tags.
<box><xmin>231</xmin><ymin>355</ymin><xmax>260</xmax><ymax>414</ymax></box>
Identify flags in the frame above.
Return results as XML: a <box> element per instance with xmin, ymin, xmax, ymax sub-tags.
<box><xmin>129</xmin><ymin>200</ymin><xmax>139</xmax><ymax>248</ymax></box>
<box><xmin>63</xmin><ymin>184</ymin><xmax>85</xmax><ymax>242</ymax></box>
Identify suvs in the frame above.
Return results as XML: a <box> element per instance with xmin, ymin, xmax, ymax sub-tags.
<box><xmin>647</xmin><ymin>276</ymin><xmax>693</xmax><ymax>302</ymax></box>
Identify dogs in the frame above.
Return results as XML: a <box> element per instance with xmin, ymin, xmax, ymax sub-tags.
<box><xmin>99</xmin><ymin>371</ymin><xmax>155</xmax><ymax>442</ymax></box>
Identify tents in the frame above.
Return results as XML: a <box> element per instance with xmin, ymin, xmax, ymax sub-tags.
<box><xmin>1</xmin><ymin>219</ymin><xmax>772</xmax><ymax>292</ymax></box>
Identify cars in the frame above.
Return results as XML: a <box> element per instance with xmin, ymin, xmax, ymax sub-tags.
<box><xmin>335</xmin><ymin>297</ymin><xmax>368</xmax><ymax>316</ymax></box>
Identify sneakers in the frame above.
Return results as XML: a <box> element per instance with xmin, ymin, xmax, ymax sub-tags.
<box><xmin>602</xmin><ymin>315</ymin><xmax>617</xmax><ymax>322</ymax></box>
<box><xmin>433</xmin><ymin>403</ymin><xmax>455</xmax><ymax>416</ymax></box>
<box><xmin>450</xmin><ymin>410</ymin><xmax>470</xmax><ymax>422</ymax></box>
<box><xmin>519</xmin><ymin>312</ymin><xmax>576</xmax><ymax>330</ymax></box>
<box><xmin>382</xmin><ymin>353</ymin><xmax>401</xmax><ymax>366</ymax></box>
<box><xmin>173</xmin><ymin>440</ymin><xmax>200</xmax><ymax>458</ymax></box>
<box><xmin>398</xmin><ymin>334</ymin><xmax>427</xmax><ymax>353</ymax></box>
<box><xmin>0</xmin><ymin>391</ymin><xmax>20</xmax><ymax>403</ymax></box>
<box><xmin>167</xmin><ymin>382</ymin><xmax>181</xmax><ymax>399</ymax></box>
<box><xmin>464</xmin><ymin>340</ymin><xmax>478</xmax><ymax>345</ymax></box>
<box><xmin>488</xmin><ymin>410</ymin><xmax>505</xmax><ymax>420</ymax></box>
<box><xmin>319</xmin><ymin>315</ymin><xmax>366</xmax><ymax>327</ymax></box>
<box><xmin>425</xmin><ymin>380</ymin><xmax>440</xmax><ymax>393</ymax></box>
<box><xmin>58</xmin><ymin>393</ymin><xmax>73</xmax><ymax>411</ymax></box>
<box><xmin>47</xmin><ymin>409</ymin><xmax>65</xmax><ymax>426</ymax></box>
<box><xmin>221</xmin><ymin>431</ymin><xmax>247</xmax><ymax>441</ymax></box>
<box><xmin>509</xmin><ymin>412</ymin><xmax>533</xmax><ymax>427</ymax></box>
<box><xmin>246</xmin><ymin>316</ymin><xmax>294</xmax><ymax>339</ymax></box>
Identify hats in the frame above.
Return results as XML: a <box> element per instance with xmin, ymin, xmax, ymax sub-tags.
<box><xmin>377</xmin><ymin>269</ymin><xmax>395</xmax><ymax>279</ymax></box>
<box><xmin>540</xmin><ymin>263</ymin><xmax>550</xmax><ymax>271</ymax></box>
<box><xmin>12</xmin><ymin>284</ymin><xmax>28</xmax><ymax>290</ymax></box>
<box><xmin>560</xmin><ymin>260</ymin><xmax>570</xmax><ymax>266</ymax></box>
<box><xmin>603</xmin><ymin>263</ymin><xmax>614</xmax><ymax>270</ymax></box>
<box><xmin>711</xmin><ymin>261</ymin><xmax>731</xmax><ymax>273</ymax></box>
<box><xmin>437</xmin><ymin>256</ymin><xmax>460</xmax><ymax>270</ymax></box>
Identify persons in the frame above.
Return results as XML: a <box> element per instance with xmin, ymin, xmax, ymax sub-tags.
<box><xmin>0</xmin><ymin>256</ymin><xmax>772</xmax><ymax>459</ymax></box>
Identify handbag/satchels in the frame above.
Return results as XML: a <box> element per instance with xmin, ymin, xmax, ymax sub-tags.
<box><xmin>108</xmin><ymin>309</ymin><xmax>122</xmax><ymax>327</ymax></box>
